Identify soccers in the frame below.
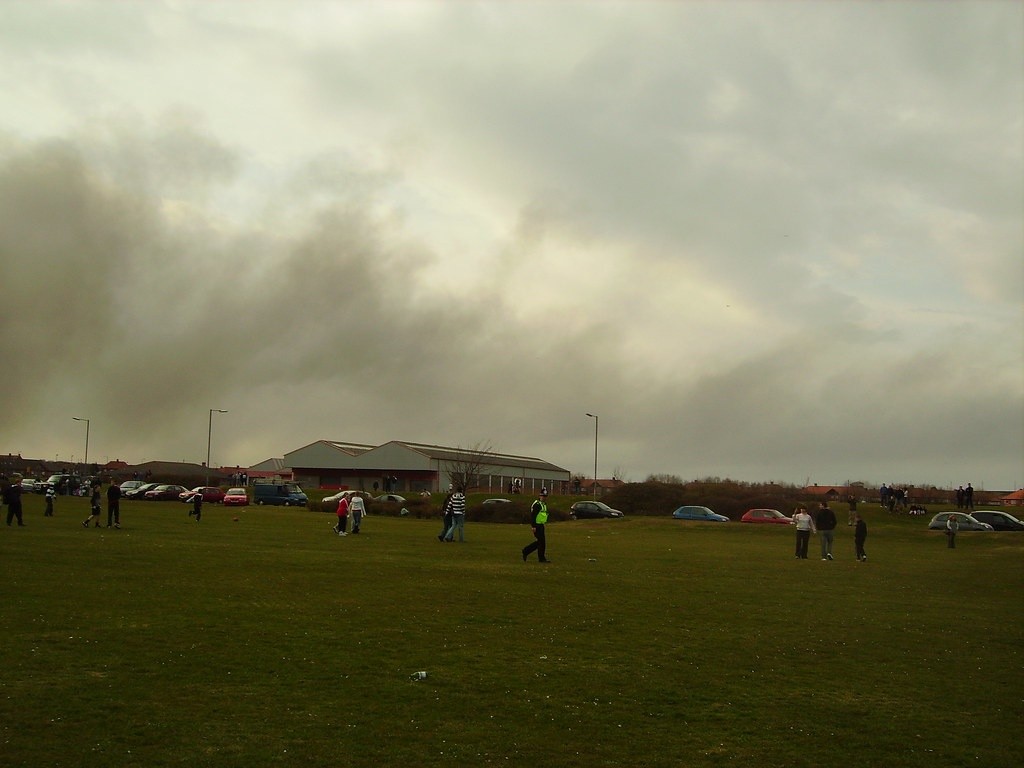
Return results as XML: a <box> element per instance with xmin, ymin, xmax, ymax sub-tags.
<box><xmin>233</xmin><ymin>517</ymin><xmax>238</xmax><ymax>521</ymax></box>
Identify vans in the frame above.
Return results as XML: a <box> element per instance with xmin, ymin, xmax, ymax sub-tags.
<box><xmin>253</xmin><ymin>479</ymin><xmax>309</xmax><ymax>508</ymax></box>
<box><xmin>46</xmin><ymin>474</ymin><xmax>82</xmax><ymax>496</ymax></box>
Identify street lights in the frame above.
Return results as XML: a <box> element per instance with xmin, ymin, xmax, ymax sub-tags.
<box><xmin>207</xmin><ymin>408</ymin><xmax>228</xmax><ymax>486</ymax></box>
<box><xmin>586</xmin><ymin>413</ymin><xmax>598</xmax><ymax>500</ymax></box>
<box><xmin>73</xmin><ymin>417</ymin><xmax>89</xmax><ymax>475</ymax></box>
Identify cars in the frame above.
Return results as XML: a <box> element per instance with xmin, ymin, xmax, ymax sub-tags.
<box><xmin>570</xmin><ymin>501</ymin><xmax>624</xmax><ymax>521</ymax></box>
<box><xmin>33</xmin><ymin>482</ymin><xmax>49</xmax><ymax>495</ymax></box>
<box><xmin>483</xmin><ymin>498</ymin><xmax>511</xmax><ymax>505</ymax></box>
<box><xmin>223</xmin><ymin>488</ymin><xmax>250</xmax><ymax>506</ymax></box>
<box><xmin>321</xmin><ymin>490</ymin><xmax>371</xmax><ymax>502</ymax></box>
<box><xmin>672</xmin><ymin>506</ymin><xmax>730</xmax><ymax>522</ymax></box>
<box><xmin>145</xmin><ymin>485</ymin><xmax>185</xmax><ymax>501</ymax></box>
<box><xmin>741</xmin><ymin>509</ymin><xmax>795</xmax><ymax>525</ymax></box>
<box><xmin>178</xmin><ymin>487</ymin><xmax>226</xmax><ymax>504</ymax></box>
<box><xmin>968</xmin><ymin>511</ymin><xmax>1024</xmax><ymax>532</ymax></box>
<box><xmin>119</xmin><ymin>481</ymin><xmax>147</xmax><ymax>498</ymax></box>
<box><xmin>928</xmin><ymin>512</ymin><xmax>994</xmax><ymax>532</ymax></box>
<box><xmin>21</xmin><ymin>479</ymin><xmax>36</xmax><ymax>492</ymax></box>
<box><xmin>125</xmin><ymin>483</ymin><xmax>163</xmax><ymax>500</ymax></box>
<box><xmin>373</xmin><ymin>494</ymin><xmax>405</xmax><ymax>502</ymax></box>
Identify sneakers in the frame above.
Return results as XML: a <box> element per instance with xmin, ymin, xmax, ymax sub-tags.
<box><xmin>827</xmin><ymin>553</ymin><xmax>833</xmax><ymax>560</ymax></box>
<box><xmin>333</xmin><ymin>526</ymin><xmax>338</xmax><ymax>533</ymax></box>
<box><xmin>821</xmin><ymin>557</ymin><xmax>827</xmax><ymax>561</ymax></box>
<box><xmin>856</xmin><ymin>559</ymin><xmax>860</xmax><ymax>562</ymax></box>
<box><xmin>114</xmin><ymin>523</ymin><xmax>121</xmax><ymax>529</ymax></box>
<box><xmin>339</xmin><ymin>531</ymin><xmax>348</xmax><ymax>537</ymax></box>
<box><xmin>862</xmin><ymin>554</ymin><xmax>867</xmax><ymax>562</ymax></box>
<box><xmin>95</xmin><ymin>523</ymin><xmax>100</xmax><ymax>528</ymax></box>
<box><xmin>107</xmin><ymin>525</ymin><xmax>112</xmax><ymax>529</ymax></box>
<box><xmin>82</xmin><ymin>522</ymin><xmax>88</xmax><ymax>527</ymax></box>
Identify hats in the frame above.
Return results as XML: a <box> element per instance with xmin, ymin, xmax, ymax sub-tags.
<box><xmin>539</xmin><ymin>486</ymin><xmax>548</xmax><ymax>496</ymax></box>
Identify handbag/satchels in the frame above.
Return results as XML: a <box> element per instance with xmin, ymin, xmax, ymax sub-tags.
<box><xmin>945</xmin><ymin>528</ymin><xmax>956</xmax><ymax>535</ymax></box>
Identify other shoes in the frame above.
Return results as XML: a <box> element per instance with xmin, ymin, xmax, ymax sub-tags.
<box><xmin>18</xmin><ymin>524</ymin><xmax>27</xmax><ymax>527</ymax></box>
<box><xmin>7</xmin><ymin>523</ymin><xmax>11</xmax><ymax>527</ymax></box>
<box><xmin>189</xmin><ymin>510</ymin><xmax>192</xmax><ymax>517</ymax></box>
<box><xmin>195</xmin><ymin>519</ymin><xmax>199</xmax><ymax>523</ymax></box>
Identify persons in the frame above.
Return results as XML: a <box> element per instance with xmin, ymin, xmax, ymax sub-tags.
<box><xmin>44</xmin><ymin>485</ymin><xmax>57</xmax><ymax>516</ymax></box>
<box><xmin>792</xmin><ymin>502</ymin><xmax>837</xmax><ymax>562</ymax></box>
<box><xmin>107</xmin><ymin>478</ymin><xmax>122</xmax><ymax>530</ymax></box>
<box><xmin>847</xmin><ymin>492</ymin><xmax>859</xmax><ymax>526</ymax></box>
<box><xmin>231</xmin><ymin>470</ymin><xmax>247</xmax><ymax>487</ymax></box>
<box><xmin>400</xmin><ymin>505</ymin><xmax>410</xmax><ymax>518</ymax></box>
<box><xmin>82</xmin><ymin>485</ymin><xmax>102</xmax><ymax>528</ymax></box>
<box><xmin>946</xmin><ymin>513</ymin><xmax>959</xmax><ymax>549</ymax></box>
<box><xmin>854</xmin><ymin>514</ymin><xmax>867</xmax><ymax>562</ymax></box>
<box><xmin>880</xmin><ymin>482</ymin><xmax>929</xmax><ymax>516</ymax></box>
<box><xmin>416</xmin><ymin>488</ymin><xmax>431</xmax><ymax>499</ymax></box>
<box><xmin>438</xmin><ymin>484</ymin><xmax>466</xmax><ymax>544</ymax></box>
<box><xmin>521</xmin><ymin>486</ymin><xmax>552</xmax><ymax>563</ymax></box>
<box><xmin>64</xmin><ymin>476</ymin><xmax>102</xmax><ymax>497</ymax></box>
<box><xmin>188</xmin><ymin>489</ymin><xmax>203</xmax><ymax>522</ymax></box>
<box><xmin>507</xmin><ymin>475</ymin><xmax>522</xmax><ymax>494</ymax></box>
<box><xmin>385</xmin><ymin>474</ymin><xmax>399</xmax><ymax>494</ymax></box>
<box><xmin>6</xmin><ymin>479</ymin><xmax>27</xmax><ymax>527</ymax></box>
<box><xmin>574</xmin><ymin>476</ymin><xmax>581</xmax><ymax>496</ymax></box>
<box><xmin>373</xmin><ymin>480</ymin><xmax>379</xmax><ymax>492</ymax></box>
<box><xmin>332</xmin><ymin>490</ymin><xmax>366</xmax><ymax>536</ymax></box>
<box><xmin>957</xmin><ymin>483</ymin><xmax>975</xmax><ymax>511</ymax></box>
